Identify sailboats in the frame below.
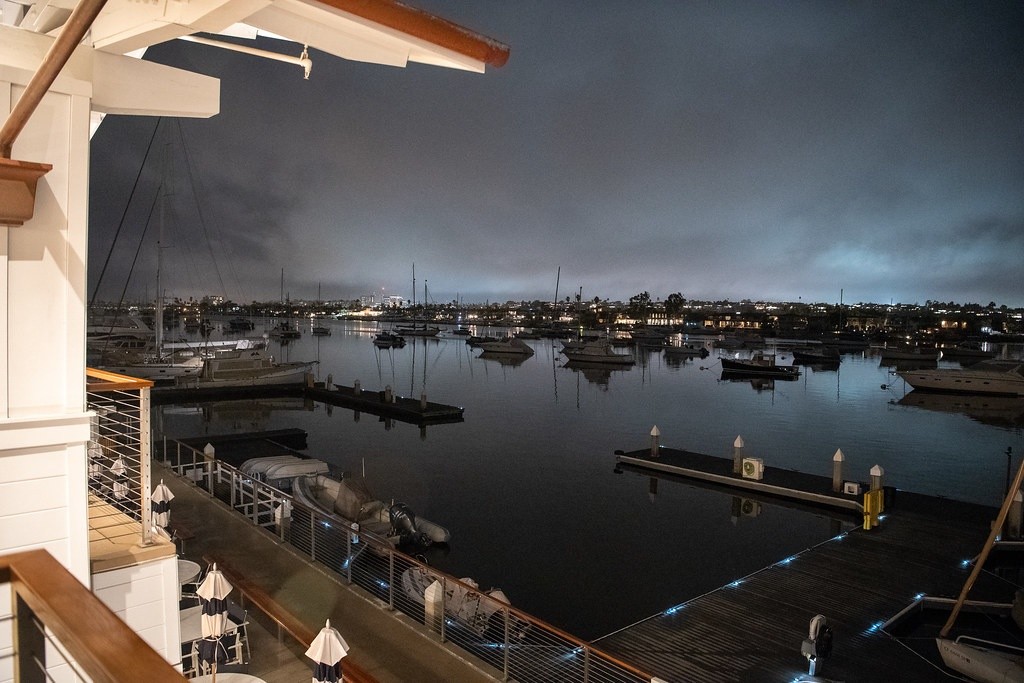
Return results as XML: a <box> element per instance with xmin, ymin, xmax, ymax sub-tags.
<box><xmin>308</xmin><ymin>282</ymin><xmax>333</xmax><ymax>335</ymax></box>
<box><xmin>935</xmin><ymin>460</ymin><xmax>1023</xmax><ymax>683</ymax></box>
<box><xmin>820</xmin><ymin>289</ymin><xmax>870</xmax><ymax>352</ymax></box>
<box><xmin>264</xmin><ymin>267</ymin><xmax>302</xmax><ymax>337</ymax></box>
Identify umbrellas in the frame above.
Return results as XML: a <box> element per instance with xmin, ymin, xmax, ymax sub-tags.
<box><xmin>196</xmin><ymin>563</ymin><xmax>233</xmax><ymax>683</ymax></box>
<box><xmin>305</xmin><ymin>619</ymin><xmax>350</xmax><ymax>683</ymax></box>
<box><xmin>88</xmin><ymin>444</ymin><xmax>103</xmax><ymax>494</ymax></box>
<box><xmin>151</xmin><ymin>479</ymin><xmax>175</xmax><ymax>538</ymax></box>
<box><xmin>111</xmin><ymin>455</ymin><xmax>133</xmax><ymax>511</ymax></box>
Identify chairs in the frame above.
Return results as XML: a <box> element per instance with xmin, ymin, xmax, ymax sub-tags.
<box><xmin>180</xmin><ymin>564</ymin><xmax>251</xmax><ymax>666</ymax></box>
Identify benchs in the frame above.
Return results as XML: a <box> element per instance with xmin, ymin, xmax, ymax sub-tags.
<box><xmin>172</xmin><ymin>526</ymin><xmax>195</xmax><ymax>556</ymax></box>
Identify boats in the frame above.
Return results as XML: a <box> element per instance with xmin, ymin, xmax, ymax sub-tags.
<box><xmin>401</xmin><ymin>566</ymin><xmax>529</xmax><ymax>652</ymax></box>
<box><xmin>714</xmin><ymin>329</ymin><xmax>767</xmax><ymax>348</ymax></box>
<box><xmin>880</xmin><ymin>345</ymin><xmax>944</xmax><ymax>361</ymax></box>
<box><xmin>477</xmin><ymin>351</ymin><xmax>531</xmax><ymax>369</ymax></box>
<box><xmin>790</xmin><ymin>347</ymin><xmax>844</xmax><ymax>365</ymax></box>
<box><xmin>200</xmin><ymin>319</ymin><xmax>215</xmax><ymax>333</ymax></box>
<box><xmin>561</xmin><ymin>359</ymin><xmax>634</xmax><ymax>386</ymax></box>
<box><xmin>471</xmin><ymin>336</ymin><xmax>534</xmax><ymax>354</ymax></box>
<box><xmin>392</xmin><ymin>261</ymin><xmax>671</xmax><ymax>346</ymax></box>
<box><xmin>661</xmin><ymin>346</ymin><xmax>711</xmax><ymax>356</ymax></box>
<box><xmin>229</xmin><ymin>317</ymin><xmax>255</xmax><ymax>329</ymax></box>
<box><xmin>561</xmin><ymin>339</ymin><xmax>634</xmax><ymax>363</ymax></box>
<box><xmin>718</xmin><ymin>351</ymin><xmax>802</xmax><ymax>379</ymax></box>
<box><xmin>886</xmin><ymin>353</ymin><xmax>1024</xmax><ymax>396</ymax></box>
<box><xmin>373</xmin><ymin>332</ymin><xmax>409</xmax><ymax>348</ymax></box>
<box><xmin>293</xmin><ymin>474</ymin><xmax>452</xmax><ymax>558</ymax></box>
<box><xmin>183</xmin><ymin>315</ymin><xmax>201</xmax><ymax>328</ymax></box>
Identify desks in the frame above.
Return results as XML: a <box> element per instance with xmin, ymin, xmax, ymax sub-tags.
<box><xmin>177</xmin><ymin>559</ymin><xmax>201</xmax><ymax>602</ymax></box>
